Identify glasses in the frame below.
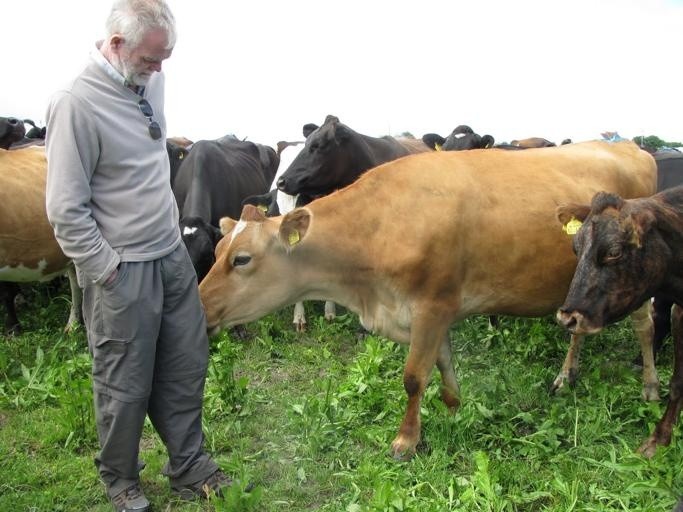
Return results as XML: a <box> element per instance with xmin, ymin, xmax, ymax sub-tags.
<box><xmin>138</xmin><ymin>99</ymin><xmax>162</xmax><ymax>140</ymax></box>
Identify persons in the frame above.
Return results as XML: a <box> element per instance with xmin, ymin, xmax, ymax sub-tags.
<box><xmin>44</xmin><ymin>0</ymin><xmax>252</xmax><ymax>512</ymax></box>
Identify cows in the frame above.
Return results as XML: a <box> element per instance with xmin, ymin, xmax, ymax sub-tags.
<box><xmin>511</xmin><ymin>137</ymin><xmax>557</xmax><ymax>150</ymax></box>
<box><xmin>169</xmin><ymin>143</ymin><xmax>276</xmax><ymax>186</ymax></box>
<box><xmin>240</xmin><ymin>141</ymin><xmax>338</xmax><ymax>332</ymax></box>
<box><xmin>0</xmin><ymin>149</ymin><xmax>85</xmax><ymax>332</ymax></box>
<box><xmin>167</xmin><ymin>135</ymin><xmax>194</xmax><ymax>149</ymax></box>
<box><xmin>553</xmin><ymin>184</ymin><xmax>683</xmax><ymax>460</ymax></box>
<box><xmin>276</xmin><ymin>115</ymin><xmax>435</xmax><ymax>207</ymax></box>
<box><xmin>650</xmin><ymin>148</ymin><xmax>683</xmax><ymax>192</ymax></box>
<box><xmin>198</xmin><ymin>141</ymin><xmax>661</xmax><ymax>464</ymax></box>
<box><xmin>172</xmin><ymin>140</ymin><xmax>269</xmax><ymax>339</ymax></box>
<box><xmin>0</xmin><ymin>116</ymin><xmax>41</xmax><ymax>148</ymax></box>
<box><xmin>423</xmin><ymin>128</ymin><xmax>527</xmax><ymax>166</ymax></box>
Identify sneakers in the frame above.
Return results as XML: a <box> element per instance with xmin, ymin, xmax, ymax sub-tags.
<box><xmin>201</xmin><ymin>468</ymin><xmax>255</xmax><ymax>498</ymax></box>
<box><xmin>111</xmin><ymin>483</ymin><xmax>153</xmax><ymax>512</ymax></box>
